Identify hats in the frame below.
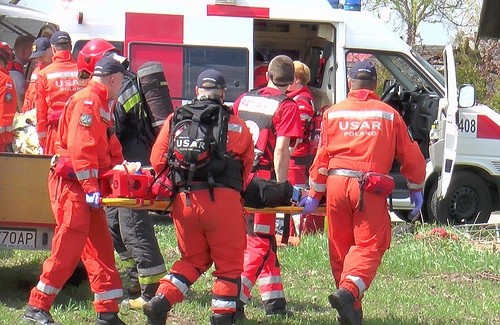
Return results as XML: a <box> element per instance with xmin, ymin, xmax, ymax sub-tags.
<box><xmin>29</xmin><ymin>37</ymin><xmax>51</xmax><ymax>60</ymax></box>
<box><xmin>93</xmin><ymin>57</ymin><xmax>134</xmax><ymax>77</ymax></box>
<box><xmin>50</xmin><ymin>31</ymin><xmax>72</xmax><ymax>44</ymax></box>
<box><xmin>197</xmin><ymin>69</ymin><xmax>228</xmax><ymax>91</ymax></box>
<box><xmin>350</xmin><ymin>61</ymin><xmax>377</xmax><ymax>81</ymax></box>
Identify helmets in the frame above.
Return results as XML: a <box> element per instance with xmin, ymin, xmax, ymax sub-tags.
<box><xmin>0</xmin><ymin>40</ymin><xmax>15</xmax><ymax>64</ymax></box>
<box><xmin>77</xmin><ymin>38</ymin><xmax>122</xmax><ymax>81</ymax></box>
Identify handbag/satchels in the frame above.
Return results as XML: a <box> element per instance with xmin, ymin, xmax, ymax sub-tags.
<box><xmin>10</xmin><ymin>108</ymin><xmax>43</xmax><ymax>156</ymax></box>
<box><xmin>362</xmin><ymin>172</ymin><xmax>395</xmax><ymax>196</ymax></box>
<box><xmin>241</xmin><ymin>177</ymin><xmax>293</xmax><ymax>209</ymax></box>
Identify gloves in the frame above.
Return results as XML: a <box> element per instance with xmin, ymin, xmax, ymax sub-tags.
<box><xmin>86</xmin><ymin>192</ymin><xmax>102</xmax><ymax>209</ymax></box>
<box><xmin>408</xmin><ymin>189</ymin><xmax>424</xmax><ymax>222</ymax></box>
<box><xmin>298</xmin><ymin>195</ymin><xmax>319</xmax><ymax>215</ymax></box>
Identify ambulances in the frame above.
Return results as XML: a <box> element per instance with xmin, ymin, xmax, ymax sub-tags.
<box><xmin>0</xmin><ymin>0</ymin><xmax>500</xmax><ymax>228</ymax></box>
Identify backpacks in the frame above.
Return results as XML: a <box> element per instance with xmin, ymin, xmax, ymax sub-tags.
<box><xmin>162</xmin><ymin>94</ymin><xmax>233</xmax><ymax>207</ymax></box>
<box><xmin>137</xmin><ymin>61</ymin><xmax>174</xmax><ymax>138</ymax></box>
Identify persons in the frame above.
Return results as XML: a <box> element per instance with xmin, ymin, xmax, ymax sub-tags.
<box><xmin>142</xmin><ymin>70</ymin><xmax>254</xmax><ymax>325</ymax></box>
<box><xmin>0</xmin><ymin>24</ymin><xmax>167</xmax><ymax>309</ymax></box>
<box><xmin>231</xmin><ymin>51</ymin><xmax>314</xmax><ymax>323</ymax></box>
<box><xmin>298</xmin><ymin>61</ymin><xmax>426</xmax><ymax>325</ymax></box>
<box><xmin>23</xmin><ymin>57</ymin><xmax>136</xmax><ymax>325</ymax></box>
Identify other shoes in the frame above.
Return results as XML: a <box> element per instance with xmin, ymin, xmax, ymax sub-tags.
<box><xmin>210</xmin><ymin>306</ymin><xmax>256</xmax><ymax>325</ymax></box>
<box><xmin>97</xmin><ymin>312</ymin><xmax>127</xmax><ymax>325</ymax></box>
<box><xmin>328</xmin><ymin>288</ymin><xmax>361</xmax><ymax>325</ymax></box>
<box><xmin>22</xmin><ymin>304</ymin><xmax>64</xmax><ymax>325</ymax></box>
<box><xmin>127</xmin><ymin>286</ymin><xmax>148</xmax><ymax>309</ymax></box>
<box><xmin>266</xmin><ymin>308</ymin><xmax>294</xmax><ymax>318</ymax></box>
<box><xmin>142</xmin><ymin>296</ymin><xmax>171</xmax><ymax>325</ymax></box>
